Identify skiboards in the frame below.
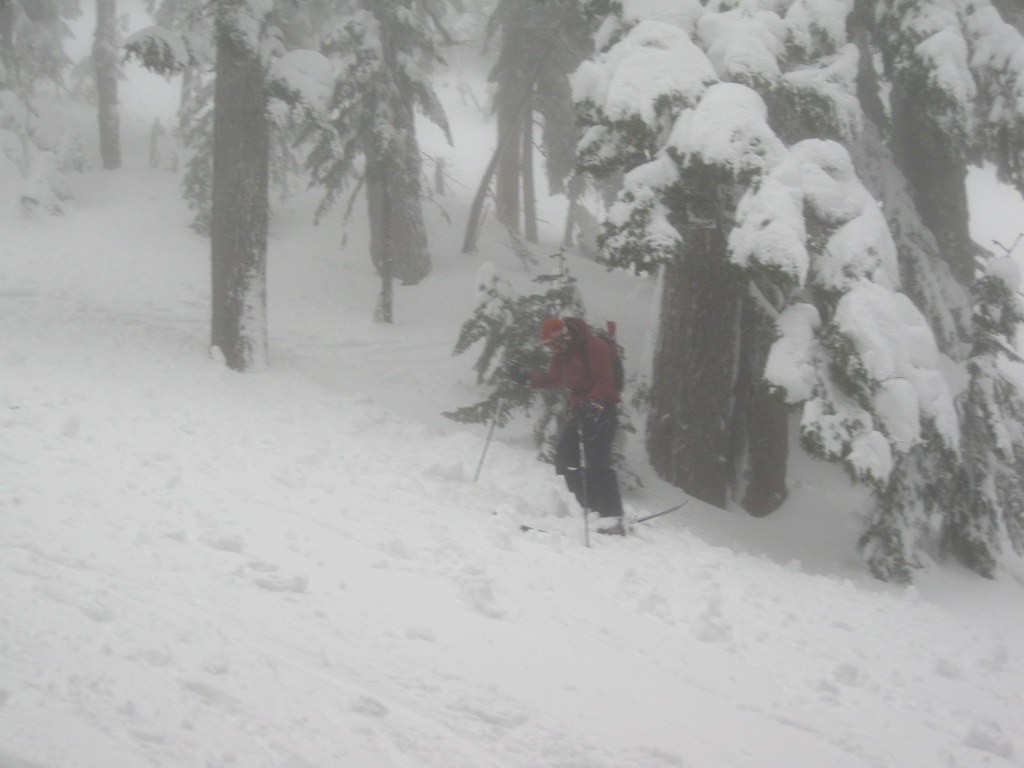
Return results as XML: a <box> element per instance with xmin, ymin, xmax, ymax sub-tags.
<box><xmin>520</xmin><ymin>498</ymin><xmax>690</xmax><ymax>533</ymax></box>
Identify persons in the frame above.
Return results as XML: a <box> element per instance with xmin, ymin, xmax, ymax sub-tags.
<box><xmin>529</xmin><ymin>317</ymin><xmax>625</xmax><ymax>535</ymax></box>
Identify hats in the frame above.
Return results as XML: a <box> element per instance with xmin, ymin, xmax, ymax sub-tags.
<box><xmin>541</xmin><ymin>318</ymin><xmax>572</xmax><ymax>345</ymax></box>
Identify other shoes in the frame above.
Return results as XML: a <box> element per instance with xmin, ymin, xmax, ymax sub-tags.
<box><xmin>598</xmin><ymin>517</ymin><xmax>626</xmax><ymax>535</ymax></box>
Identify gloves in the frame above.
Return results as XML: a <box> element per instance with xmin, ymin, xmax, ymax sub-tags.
<box><xmin>582</xmin><ymin>402</ymin><xmax>604</xmax><ymax>422</ymax></box>
